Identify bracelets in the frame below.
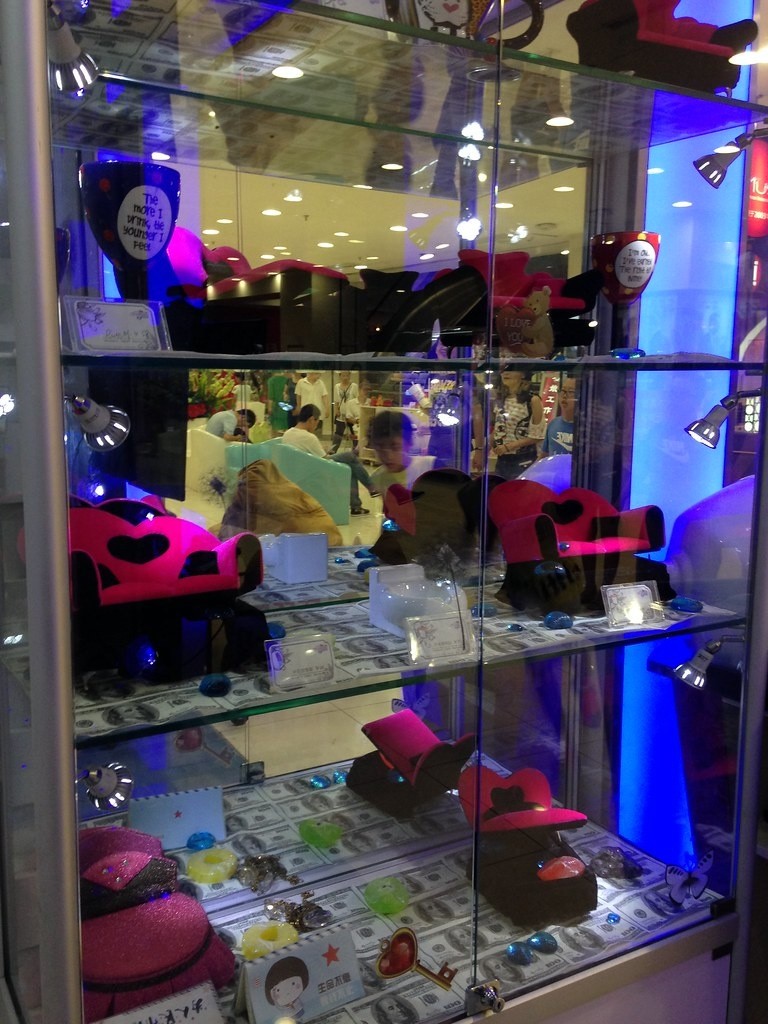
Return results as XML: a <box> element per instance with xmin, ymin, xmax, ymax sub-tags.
<box><xmin>475</xmin><ymin>447</ymin><xmax>483</xmax><ymax>450</ymax></box>
<box><xmin>504</xmin><ymin>445</ymin><xmax>510</xmax><ymax>452</ymax></box>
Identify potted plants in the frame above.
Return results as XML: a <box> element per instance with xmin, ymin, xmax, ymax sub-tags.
<box><xmin>189</xmin><ymin>369</ymin><xmax>238</xmax><ymax>418</ymax></box>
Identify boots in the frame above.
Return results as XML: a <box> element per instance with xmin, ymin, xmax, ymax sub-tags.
<box><xmin>328</xmin><ymin>434</ymin><xmax>342</xmax><ymax>454</ymax></box>
<box><xmin>351</xmin><ymin>439</ymin><xmax>358</xmax><ymax>452</ymax></box>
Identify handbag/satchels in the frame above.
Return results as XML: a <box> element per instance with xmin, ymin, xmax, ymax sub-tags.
<box><xmin>334</xmin><ymin>415</ymin><xmax>338</xmax><ymax>424</ymax></box>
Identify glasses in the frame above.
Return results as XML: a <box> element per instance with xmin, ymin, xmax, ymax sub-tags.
<box><xmin>557</xmin><ymin>388</ymin><xmax>576</xmax><ymax>397</ymax></box>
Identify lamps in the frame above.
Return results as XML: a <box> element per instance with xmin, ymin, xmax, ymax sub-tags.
<box><xmin>75</xmin><ymin>761</ymin><xmax>134</xmax><ymax>809</ymax></box>
<box><xmin>693</xmin><ymin>129</ymin><xmax>768</xmax><ymax>189</ymax></box>
<box><xmin>684</xmin><ymin>389</ymin><xmax>760</xmax><ymax>449</ymax></box>
<box><xmin>63</xmin><ymin>393</ymin><xmax>130</xmax><ymax>451</ymax></box>
<box><xmin>46</xmin><ymin>0</ymin><xmax>100</xmax><ymax>92</ymax></box>
<box><xmin>674</xmin><ymin>634</ymin><xmax>747</xmax><ymax>691</ymax></box>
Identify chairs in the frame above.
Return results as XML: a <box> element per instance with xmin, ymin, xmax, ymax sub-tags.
<box><xmin>268</xmin><ymin>443</ymin><xmax>351</xmax><ymax>524</ymax></box>
<box><xmin>190</xmin><ymin>424</ymin><xmax>249</xmax><ymax>491</ymax></box>
<box><xmin>224</xmin><ymin>437</ymin><xmax>282</xmax><ymax>482</ymax></box>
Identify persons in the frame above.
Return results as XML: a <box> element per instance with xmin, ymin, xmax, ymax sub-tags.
<box><xmin>220</xmin><ymin>462</ymin><xmax>343</xmax><ymax>548</ymax></box>
<box><xmin>282</xmin><ymin>404</ymin><xmax>382</xmax><ymax>518</ymax></box>
<box><xmin>484</xmin><ymin>366</ymin><xmax>546</xmax><ymax>481</ymax></box>
<box><xmin>368</xmin><ymin>411</ymin><xmax>442</xmax><ymax>498</ymax></box>
<box><xmin>427</xmin><ymin>342</ymin><xmax>484</xmax><ymax>478</ymax></box>
<box><xmin>538</xmin><ymin>376</ymin><xmax>581</xmax><ymax>459</ymax></box>
<box><xmin>232</xmin><ymin>369</ymin><xmax>305</xmax><ymax>432</ymax></box>
<box><xmin>207</xmin><ymin>407</ymin><xmax>256</xmax><ymax>444</ymax></box>
<box><xmin>325</xmin><ymin>370</ymin><xmax>358</xmax><ymax>454</ymax></box>
<box><xmin>293</xmin><ymin>371</ymin><xmax>330</xmax><ymax>433</ymax></box>
<box><xmin>346</xmin><ymin>380</ymin><xmax>372</xmax><ymax>451</ymax></box>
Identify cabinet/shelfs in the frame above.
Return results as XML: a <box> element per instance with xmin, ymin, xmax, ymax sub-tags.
<box><xmin>0</xmin><ymin>0</ymin><xmax>768</xmax><ymax>1024</ymax></box>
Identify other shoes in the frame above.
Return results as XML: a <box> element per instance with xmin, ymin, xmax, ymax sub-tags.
<box><xmin>371</xmin><ymin>488</ymin><xmax>384</xmax><ymax>498</ymax></box>
<box><xmin>351</xmin><ymin>506</ymin><xmax>370</xmax><ymax>517</ymax></box>
